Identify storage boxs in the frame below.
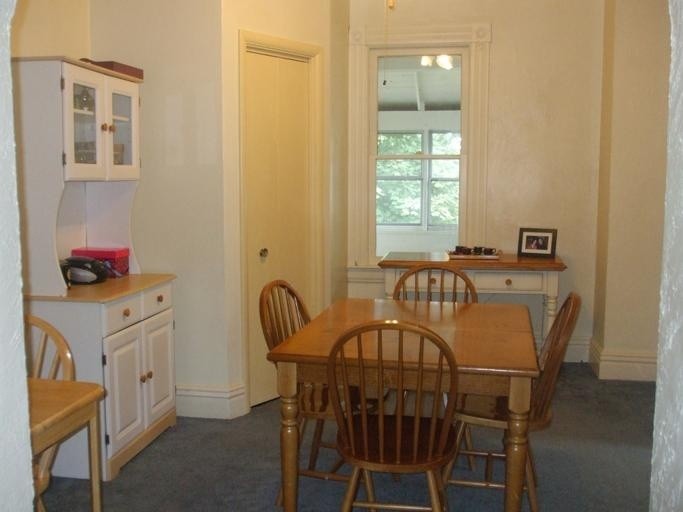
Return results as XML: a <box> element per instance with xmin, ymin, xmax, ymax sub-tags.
<box><xmin>71</xmin><ymin>248</ymin><xmax>130</xmax><ymax>279</ymax></box>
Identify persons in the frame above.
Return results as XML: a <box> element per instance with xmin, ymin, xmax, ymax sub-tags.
<box><xmin>526</xmin><ymin>238</ymin><xmax>538</xmax><ymax>249</ymax></box>
<box><xmin>536</xmin><ymin>237</ymin><xmax>546</xmax><ymax>249</ymax></box>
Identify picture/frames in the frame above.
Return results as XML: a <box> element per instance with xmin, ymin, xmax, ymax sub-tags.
<box><xmin>518</xmin><ymin>228</ymin><xmax>557</xmax><ymax>259</ymax></box>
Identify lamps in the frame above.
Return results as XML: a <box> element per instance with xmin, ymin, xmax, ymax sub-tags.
<box><xmin>420</xmin><ymin>55</ymin><xmax>453</xmax><ymax>70</ymax></box>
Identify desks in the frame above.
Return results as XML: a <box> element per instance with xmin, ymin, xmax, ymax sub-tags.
<box><xmin>27</xmin><ymin>377</ymin><xmax>108</xmax><ymax>511</ymax></box>
<box><xmin>266</xmin><ymin>298</ymin><xmax>541</xmax><ymax>512</ymax></box>
<box><xmin>377</xmin><ymin>251</ymin><xmax>568</xmax><ymax>336</ymax></box>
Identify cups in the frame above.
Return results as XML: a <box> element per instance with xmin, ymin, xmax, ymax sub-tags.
<box><xmin>113</xmin><ymin>144</ymin><xmax>124</xmax><ymax>164</ymax></box>
<box><xmin>75</xmin><ymin>141</ymin><xmax>96</xmax><ymax>164</ymax></box>
<box><xmin>456</xmin><ymin>246</ymin><xmax>497</xmax><ymax>256</ymax></box>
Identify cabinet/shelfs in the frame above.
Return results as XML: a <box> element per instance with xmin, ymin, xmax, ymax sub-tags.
<box><xmin>10</xmin><ymin>57</ymin><xmax>141</xmax><ymax>181</ymax></box>
<box><xmin>22</xmin><ymin>274</ymin><xmax>177</xmax><ymax>483</ymax></box>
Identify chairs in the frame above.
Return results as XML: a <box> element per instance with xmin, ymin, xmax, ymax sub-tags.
<box><xmin>21</xmin><ymin>314</ymin><xmax>76</xmax><ymax>511</ymax></box>
<box><xmin>328</xmin><ymin>320</ymin><xmax>459</xmax><ymax>512</ymax></box>
<box><xmin>393</xmin><ymin>261</ymin><xmax>478</xmax><ymax>414</ymax></box>
<box><xmin>259</xmin><ymin>279</ymin><xmax>391</xmax><ymax>506</ymax></box>
<box><xmin>439</xmin><ymin>291</ymin><xmax>581</xmax><ymax>512</ymax></box>
